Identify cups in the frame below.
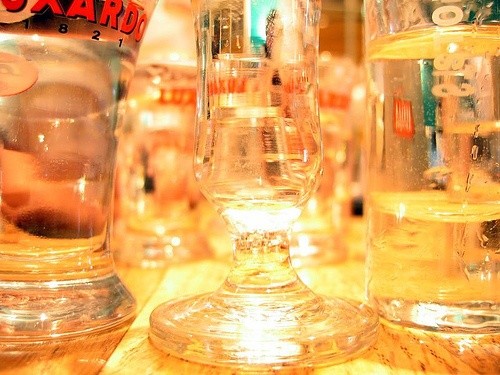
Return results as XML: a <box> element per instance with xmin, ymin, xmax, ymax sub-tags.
<box><xmin>110</xmin><ymin>63</ymin><xmax>205</xmax><ymax>268</ymax></box>
<box><xmin>360</xmin><ymin>0</ymin><xmax>500</xmax><ymax>334</ymax></box>
<box><xmin>0</xmin><ymin>0</ymin><xmax>160</xmax><ymax>347</ymax></box>
<box><xmin>287</xmin><ymin>60</ymin><xmax>373</xmax><ymax>265</ymax></box>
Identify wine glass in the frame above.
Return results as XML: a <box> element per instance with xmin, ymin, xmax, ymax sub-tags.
<box><xmin>147</xmin><ymin>0</ymin><xmax>380</xmax><ymax>370</ymax></box>
